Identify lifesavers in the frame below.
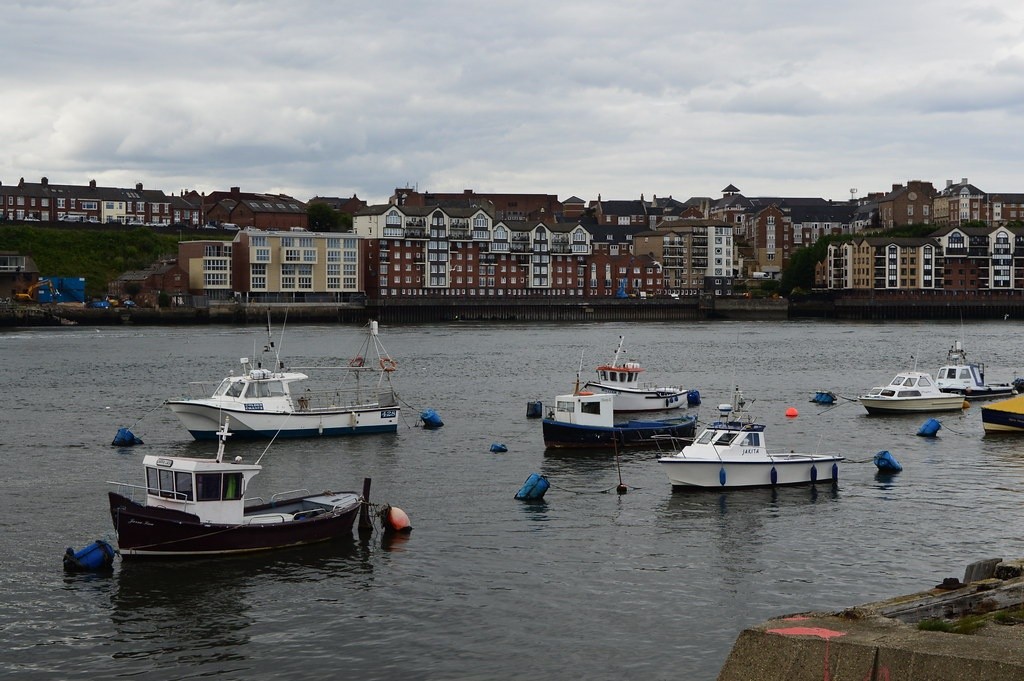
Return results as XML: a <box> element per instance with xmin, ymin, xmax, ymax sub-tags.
<box><xmin>579</xmin><ymin>391</ymin><xmax>592</xmax><ymax>396</ymax></box>
<box><xmin>351</xmin><ymin>356</ymin><xmax>363</xmax><ymax>367</ymax></box>
<box><xmin>378</xmin><ymin>357</ymin><xmax>397</xmax><ymax>371</ymax></box>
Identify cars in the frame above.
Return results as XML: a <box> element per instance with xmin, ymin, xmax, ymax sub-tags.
<box><xmin>85</xmin><ymin>293</ymin><xmax>136</xmax><ymax>309</ymax></box>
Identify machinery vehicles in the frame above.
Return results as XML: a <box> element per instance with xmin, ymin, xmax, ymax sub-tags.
<box><xmin>14</xmin><ymin>279</ymin><xmax>59</xmax><ymax>304</ymax></box>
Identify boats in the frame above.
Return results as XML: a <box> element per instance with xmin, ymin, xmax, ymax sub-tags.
<box><xmin>979</xmin><ymin>393</ymin><xmax>1024</xmax><ymax>432</ymax></box>
<box><xmin>104</xmin><ymin>391</ymin><xmax>361</xmax><ymax>560</ymax></box>
<box><xmin>932</xmin><ymin>310</ymin><xmax>1015</xmax><ymax>399</ymax></box>
<box><xmin>540</xmin><ymin>346</ymin><xmax>699</xmax><ymax>450</ymax></box>
<box><xmin>649</xmin><ymin>333</ymin><xmax>847</xmax><ymax>490</ymax></box>
<box><xmin>856</xmin><ymin>344</ymin><xmax>966</xmax><ymax>415</ymax></box>
<box><xmin>582</xmin><ymin>334</ymin><xmax>690</xmax><ymax>412</ymax></box>
<box><xmin>162</xmin><ymin>301</ymin><xmax>403</xmax><ymax>441</ymax></box>
